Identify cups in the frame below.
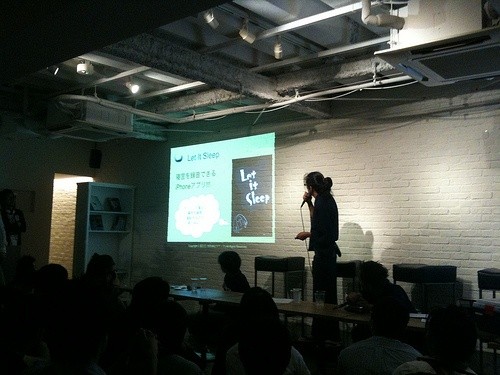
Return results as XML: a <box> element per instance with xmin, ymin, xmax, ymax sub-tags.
<box><xmin>315</xmin><ymin>291</ymin><xmax>325</xmax><ymax>306</ymax></box>
<box><xmin>191</xmin><ymin>278</ymin><xmax>198</xmax><ymax>292</ymax></box>
<box><xmin>292</xmin><ymin>288</ymin><xmax>302</xmax><ymax>303</ymax></box>
<box><xmin>200</xmin><ymin>278</ymin><xmax>207</xmax><ymax>291</ymax></box>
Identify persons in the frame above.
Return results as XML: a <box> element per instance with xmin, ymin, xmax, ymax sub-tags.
<box><xmin>0</xmin><ymin>215</ymin><xmax>311</xmax><ymax>375</ymax></box>
<box><xmin>295</xmin><ymin>172</ymin><xmax>341</xmax><ymax>339</ymax></box>
<box><xmin>393</xmin><ymin>309</ymin><xmax>478</xmax><ymax>375</ymax></box>
<box><xmin>346</xmin><ymin>261</ymin><xmax>392</xmax><ymax>343</ymax></box>
<box><xmin>337</xmin><ymin>285</ymin><xmax>423</xmax><ymax>375</ymax></box>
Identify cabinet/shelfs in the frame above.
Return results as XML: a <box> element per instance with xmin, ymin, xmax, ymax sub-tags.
<box><xmin>72</xmin><ymin>182</ymin><xmax>137</xmax><ymax>289</ymax></box>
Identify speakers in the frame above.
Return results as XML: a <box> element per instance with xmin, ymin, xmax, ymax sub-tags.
<box><xmin>90</xmin><ymin>149</ymin><xmax>102</xmax><ymax>169</ymax></box>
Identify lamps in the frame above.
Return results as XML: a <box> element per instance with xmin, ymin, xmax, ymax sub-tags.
<box><xmin>89</xmin><ymin>143</ymin><xmax>102</xmax><ymax>169</ymax></box>
<box><xmin>202</xmin><ymin>8</ymin><xmax>219</xmax><ymax>30</ymax></box>
<box><xmin>273</xmin><ymin>35</ymin><xmax>282</xmax><ymax>59</ymax></box>
<box><xmin>125</xmin><ymin>76</ymin><xmax>140</xmax><ymax>94</ymax></box>
<box><xmin>239</xmin><ymin>18</ymin><xmax>256</xmax><ymax>45</ymax></box>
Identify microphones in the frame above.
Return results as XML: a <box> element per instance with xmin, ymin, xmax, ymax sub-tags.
<box><xmin>300</xmin><ymin>193</ymin><xmax>310</xmax><ymax>207</ymax></box>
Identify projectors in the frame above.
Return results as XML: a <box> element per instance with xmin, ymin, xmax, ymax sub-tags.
<box><xmin>49</xmin><ymin>100</ymin><xmax>134</xmax><ymax>141</ymax></box>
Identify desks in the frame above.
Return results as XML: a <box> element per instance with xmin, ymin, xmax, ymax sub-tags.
<box><xmin>169</xmin><ymin>254</ymin><xmax>500</xmax><ymax>353</ymax></box>
